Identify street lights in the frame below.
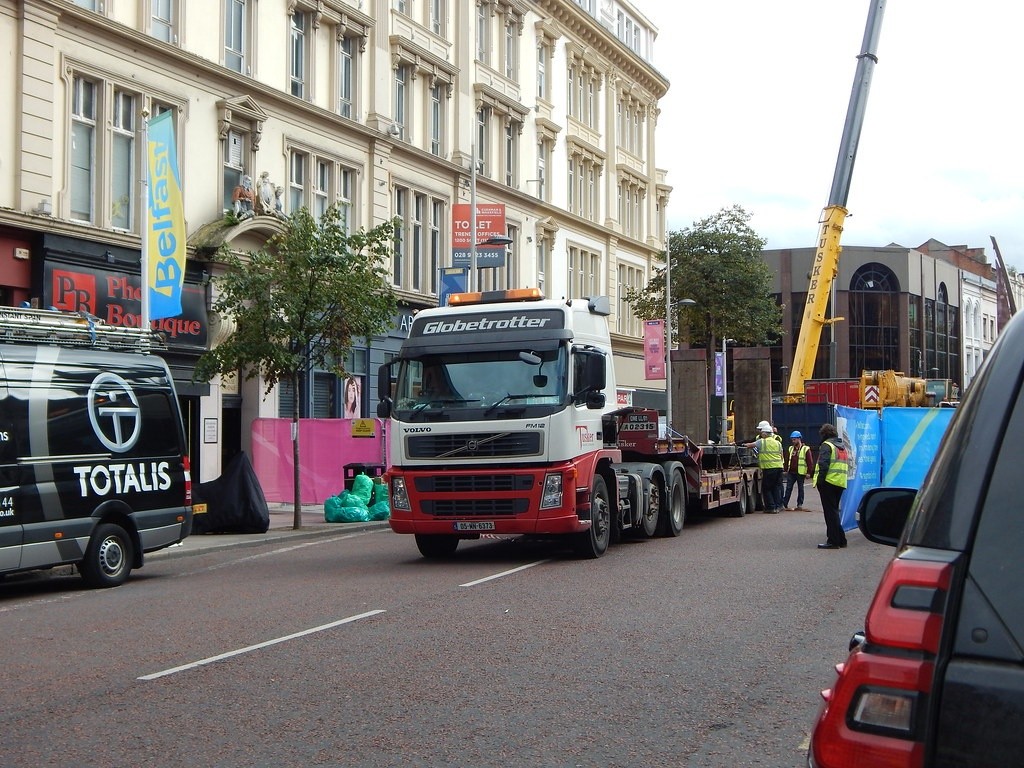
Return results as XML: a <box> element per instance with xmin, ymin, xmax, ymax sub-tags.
<box><xmin>721</xmin><ymin>337</ymin><xmax>737</xmax><ymax>440</ymax></box>
<box><xmin>663</xmin><ymin>298</ymin><xmax>697</xmax><ymax>432</ymax></box>
<box><xmin>470</xmin><ymin>144</ymin><xmax>515</xmax><ymax>294</ymax></box>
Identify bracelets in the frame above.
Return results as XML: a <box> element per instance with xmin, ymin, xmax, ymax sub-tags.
<box><xmin>742</xmin><ymin>443</ymin><xmax>744</xmax><ymax>447</ymax></box>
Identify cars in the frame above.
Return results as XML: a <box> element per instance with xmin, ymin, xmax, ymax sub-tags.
<box><xmin>805</xmin><ymin>309</ymin><xmax>1023</xmax><ymax>768</ymax></box>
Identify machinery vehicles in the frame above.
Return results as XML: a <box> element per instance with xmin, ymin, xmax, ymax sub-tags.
<box><xmin>727</xmin><ymin>1</ymin><xmax>962</xmax><ymax>450</ymax></box>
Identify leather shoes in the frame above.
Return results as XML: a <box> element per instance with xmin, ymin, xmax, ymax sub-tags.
<box><xmin>818</xmin><ymin>543</ymin><xmax>847</xmax><ymax>549</ymax></box>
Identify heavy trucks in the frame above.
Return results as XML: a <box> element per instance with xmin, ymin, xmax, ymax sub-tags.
<box><xmin>378</xmin><ymin>290</ymin><xmax>763</xmax><ymax>560</ymax></box>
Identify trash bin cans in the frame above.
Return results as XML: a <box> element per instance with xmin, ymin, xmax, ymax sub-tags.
<box><xmin>342</xmin><ymin>462</ymin><xmax>386</xmax><ymax>492</ymax></box>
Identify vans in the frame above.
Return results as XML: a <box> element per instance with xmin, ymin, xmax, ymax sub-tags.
<box><xmin>0</xmin><ymin>303</ymin><xmax>192</xmax><ymax>590</ymax></box>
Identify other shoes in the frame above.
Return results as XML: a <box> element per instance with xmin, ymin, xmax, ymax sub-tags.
<box><xmin>763</xmin><ymin>508</ymin><xmax>779</xmax><ymax>513</ymax></box>
<box><xmin>797</xmin><ymin>504</ymin><xmax>802</xmax><ymax>508</ymax></box>
<box><xmin>783</xmin><ymin>505</ymin><xmax>787</xmax><ymax>508</ymax></box>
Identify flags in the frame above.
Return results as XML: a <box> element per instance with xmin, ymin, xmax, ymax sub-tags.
<box><xmin>147</xmin><ymin>110</ymin><xmax>187</xmax><ymax>319</ymax></box>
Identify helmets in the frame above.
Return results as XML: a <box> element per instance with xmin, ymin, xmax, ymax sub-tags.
<box><xmin>756</xmin><ymin>420</ymin><xmax>778</xmax><ymax>432</ymax></box>
<box><xmin>790</xmin><ymin>430</ymin><xmax>802</xmax><ymax>438</ymax></box>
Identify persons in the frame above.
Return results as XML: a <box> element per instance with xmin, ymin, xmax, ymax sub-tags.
<box><xmin>952</xmin><ymin>383</ymin><xmax>959</xmax><ymax>402</ymax></box>
<box><xmin>784</xmin><ymin>431</ymin><xmax>813</xmax><ymax>510</ymax></box>
<box><xmin>813</xmin><ymin>423</ymin><xmax>847</xmax><ymax>549</ymax></box>
<box><xmin>742</xmin><ymin>421</ymin><xmax>784</xmax><ymax>513</ymax></box>
<box><xmin>232</xmin><ymin>171</ymin><xmax>292</xmax><ymax>221</ymax></box>
<box><xmin>345</xmin><ymin>377</ymin><xmax>360</xmax><ymax>418</ymax></box>
